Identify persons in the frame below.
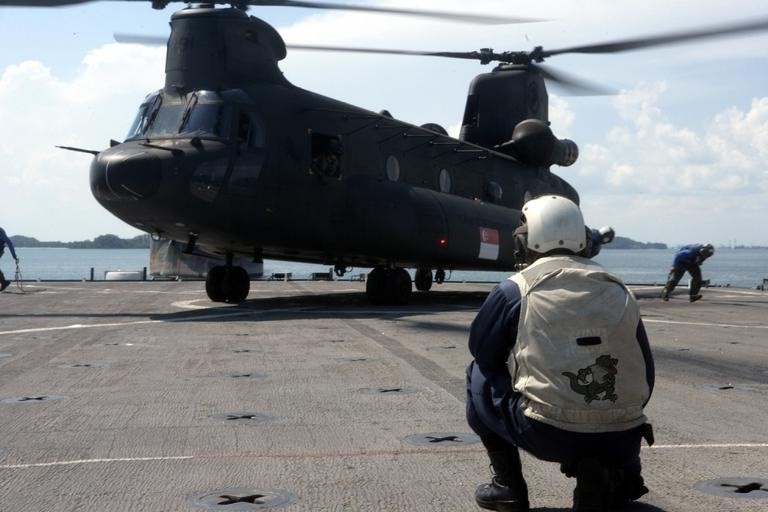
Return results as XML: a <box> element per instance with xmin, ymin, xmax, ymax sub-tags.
<box><xmin>466</xmin><ymin>195</ymin><xmax>657</xmax><ymax>512</ymax></box>
<box><xmin>659</xmin><ymin>243</ymin><xmax>716</xmax><ymax>302</ymax></box>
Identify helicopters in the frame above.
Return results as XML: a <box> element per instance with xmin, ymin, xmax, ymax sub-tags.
<box><xmin>0</xmin><ymin>0</ymin><xmax>768</xmax><ymax>306</ymax></box>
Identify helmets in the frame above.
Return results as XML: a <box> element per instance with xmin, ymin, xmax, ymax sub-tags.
<box><xmin>520</xmin><ymin>194</ymin><xmax>587</xmax><ymax>252</ymax></box>
<box><xmin>704</xmin><ymin>243</ymin><xmax>715</xmax><ymax>256</ymax></box>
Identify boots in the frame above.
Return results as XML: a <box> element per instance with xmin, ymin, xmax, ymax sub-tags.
<box><xmin>690</xmin><ymin>280</ymin><xmax>702</xmax><ymax>302</ymax></box>
<box><xmin>662</xmin><ymin>280</ymin><xmax>678</xmax><ymax>301</ymax></box>
<box><xmin>475</xmin><ymin>435</ymin><xmax>530</xmax><ymax>511</ymax></box>
